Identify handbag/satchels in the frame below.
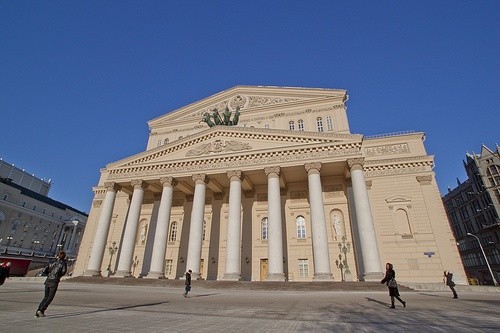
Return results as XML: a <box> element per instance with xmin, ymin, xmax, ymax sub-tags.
<box><xmin>388</xmin><ymin>277</ymin><xmax>397</xmax><ymax>287</ymax></box>
<box><xmin>185</xmin><ymin>285</ymin><xmax>191</xmax><ymax>291</ymax></box>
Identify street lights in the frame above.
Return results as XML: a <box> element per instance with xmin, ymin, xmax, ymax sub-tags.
<box><xmin>57</xmin><ymin>244</ymin><xmax>63</xmax><ymax>250</ymax></box>
<box><xmin>32</xmin><ymin>240</ymin><xmax>39</xmax><ymax>256</ymax></box>
<box><xmin>4</xmin><ymin>236</ymin><xmax>13</xmax><ymax>254</ymax></box>
<box><xmin>467</xmin><ymin>232</ymin><xmax>498</xmax><ymax>286</ymax></box>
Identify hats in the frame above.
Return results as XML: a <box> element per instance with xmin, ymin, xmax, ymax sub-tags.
<box><xmin>188</xmin><ymin>270</ymin><xmax>192</xmax><ymax>272</ymax></box>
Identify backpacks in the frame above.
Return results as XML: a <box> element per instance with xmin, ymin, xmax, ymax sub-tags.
<box><xmin>47</xmin><ymin>262</ymin><xmax>63</xmax><ymax>280</ymax></box>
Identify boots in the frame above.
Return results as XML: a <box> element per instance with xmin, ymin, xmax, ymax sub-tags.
<box><xmin>183</xmin><ymin>292</ymin><xmax>187</xmax><ymax>298</ymax></box>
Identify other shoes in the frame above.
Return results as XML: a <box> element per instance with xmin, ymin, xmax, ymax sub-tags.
<box><xmin>403</xmin><ymin>302</ymin><xmax>406</xmax><ymax>308</ymax></box>
<box><xmin>34</xmin><ymin>309</ymin><xmax>44</xmax><ymax>318</ymax></box>
<box><xmin>390</xmin><ymin>305</ymin><xmax>395</xmax><ymax>309</ymax></box>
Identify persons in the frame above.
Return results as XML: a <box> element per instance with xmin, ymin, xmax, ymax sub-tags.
<box><xmin>33</xmin><ymin>251</ymin><xmax>67</xmax><ymax>318</ymax></box>
<box><xmin>381</xmin><ymin>262</ymin><xmax>406</xmax><ymax>309</ymax></box>
<box><xmin>0</xmin><ymin>259</ymin><xmax>11</xmax><ymax>285</ymax></box>
<box><xmin>183</xmin><ymin>269</ymin><xmax>192</xmax><ymax>298</ymax></box>
<box><xmin>444</xmin><ymin>269</ymin><xmax>459</xmax><ymax>299</ymax></box>
<box><xmin>203</xmin><ymin>106</ymin><xmax>241</xmax><ymax>128</ymax></box>
